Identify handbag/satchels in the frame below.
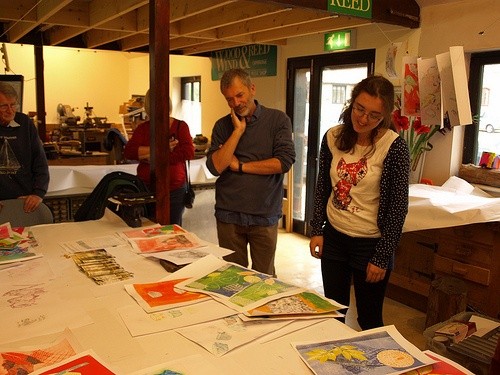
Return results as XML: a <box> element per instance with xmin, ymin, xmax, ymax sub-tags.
<box><xmin>185</xmin><ymin>189</ymin><xmax>195</xmax><ymax>209</ymax></box>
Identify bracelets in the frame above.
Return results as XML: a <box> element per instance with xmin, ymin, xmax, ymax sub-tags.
<box><xmin>238</xmin><ymin>161</ymin><xmax>243</xmax><ymax>173</ymax></box>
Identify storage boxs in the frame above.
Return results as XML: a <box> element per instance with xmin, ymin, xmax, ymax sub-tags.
<box><xmin>424</xmin><ymin>312</ymin><xmax>500</xmax><ymax>375</ymax></box>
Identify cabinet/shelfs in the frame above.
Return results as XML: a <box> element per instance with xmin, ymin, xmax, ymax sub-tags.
<box><xmin>384</xmin><ymin>220</ymin><xmax>500</xmax><ymax>321</ymax></box>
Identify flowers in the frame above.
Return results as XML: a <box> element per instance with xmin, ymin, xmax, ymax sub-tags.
<box><xmin>390</xmin><ymin>93</ymin><xmax>442</xmax><ymax>171</ymax></box>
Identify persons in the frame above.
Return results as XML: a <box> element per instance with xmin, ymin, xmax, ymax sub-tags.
<box><xmin>0</xmin><ymin>83</ymin><xmax>50</xmax><ymax>213</ymax></box>
<box><xmin>206</xmin><ymin>68</ymin><xmax>296</xmax><ymax>277</ymax></box>
<box><xmin>309</xmin><ymin>76</ymin><xmax>410</xmax><ymax>331</ymax></box>
<box><xmin>124</xmin><ymin>89</ymin><xmax>195</xmax><ymax>228</ymax></box>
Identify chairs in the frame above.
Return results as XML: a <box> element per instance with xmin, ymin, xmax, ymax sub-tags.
<box><xmin>0</xmin><ymin>199</ymin><xmax>53</xmax><ymax>228</ymax></box>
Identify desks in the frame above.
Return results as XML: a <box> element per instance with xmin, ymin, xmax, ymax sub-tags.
<box><xmin>0</xmin><ymin>207</ymin><xmax>359</xmax><ymax>375</ymax></box>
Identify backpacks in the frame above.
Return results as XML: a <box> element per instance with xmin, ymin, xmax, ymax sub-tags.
<box><xmin>74</xmin><ymin>171</ymin><xmax>145</xmax><ymax>222</ymax></box>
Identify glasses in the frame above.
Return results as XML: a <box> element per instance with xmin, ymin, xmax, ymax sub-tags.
<box><xmin>0</xmin><ymin>103</ymin><xmax>20</xmax><ymax>111</ymax></box>
<box><xmin>352</xmin><ymin>105</ymin><xmax>382</xmax><ymax>122</ymax></box>
